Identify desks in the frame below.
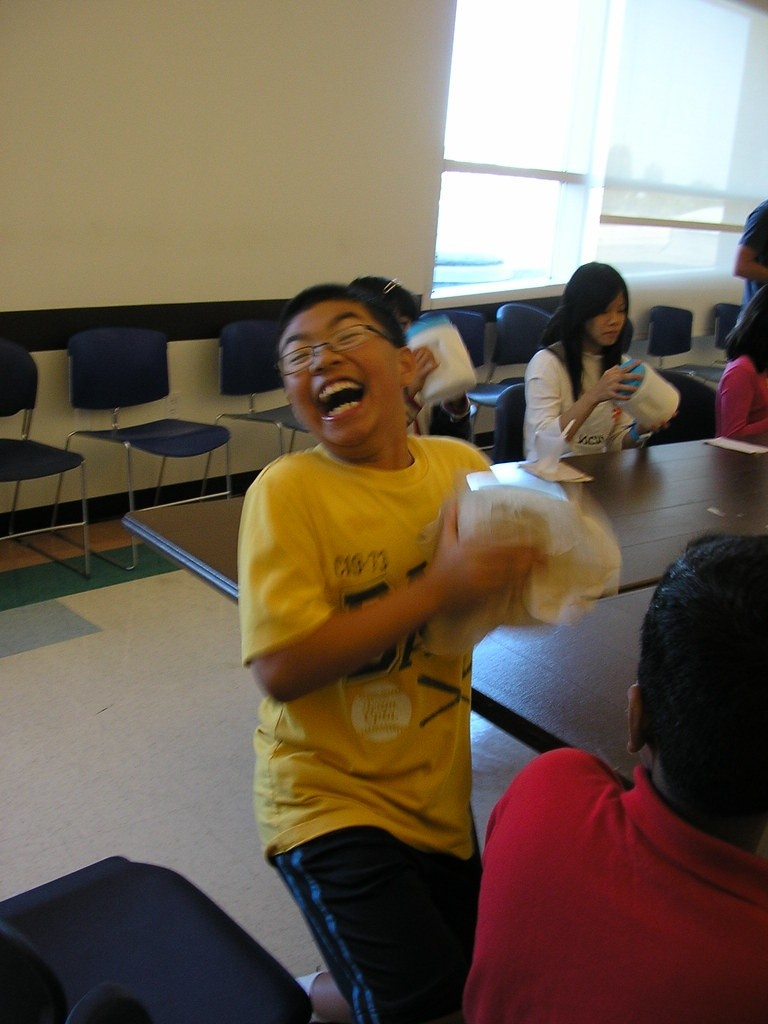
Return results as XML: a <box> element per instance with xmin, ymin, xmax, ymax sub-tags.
<box><xmin>120</xmin><ymin>437</ymin><xmax>768</xmax><ymax>605</ymax></box>
<box><xmin>470</xmin><ymin>582</ymin><xmax>661</xmax><ymax>790</ymax></box>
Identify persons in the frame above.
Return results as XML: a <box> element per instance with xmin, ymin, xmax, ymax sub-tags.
<box><xmin>715</xmin><ymin>285</ymin><xmax>768</xmax><ymax>439</ymax></box>
<box><xmin>348</xmin><ymin>277</ymin><xmax>472</xmax><ymax>442</ymax></box>
<box><xmin>237</xmin><ymin>281</ymin><xmax>567</xmax><ymax>1024</ymax></box>
<box><xmin>463</xmin><ymin>530</ymin><xmax>768</xmax><ymax>1024</ymax></box>
<box><xmin>733</xmin><ymin>199</ymin><xmax>768</xmax><ymax>305</ymax></box>
<box><xmin>523</xmin><ymin>262</ymin><xmax>678</xmax><ymax>458</ymax></box>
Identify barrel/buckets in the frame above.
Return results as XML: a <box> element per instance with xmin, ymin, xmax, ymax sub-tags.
<box><xmin>405</xmin><ymin>314</ymin><xmax>476</xmax><ymax>402</ymax></box>
<box><xmin>611</xmin><ymin>360</ymin><xmax>680</xmax><ymax>427</ymax></box>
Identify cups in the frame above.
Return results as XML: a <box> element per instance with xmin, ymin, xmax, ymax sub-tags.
<box><xmin>536</xmin><ymin>431</ymin><xmax>564</xmax><ymax>474</ymax></box>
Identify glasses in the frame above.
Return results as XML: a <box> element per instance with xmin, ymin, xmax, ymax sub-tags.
<box><xmin>272</xmin><ymin>323</ymin><xmax>398</xmax><ymax>376</ymax></box>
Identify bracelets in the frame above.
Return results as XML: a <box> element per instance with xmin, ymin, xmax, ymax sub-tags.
<box><xmin>440</xmin><ymin>401</ymin><xmax>471</xmax><ymax>420</ymax></box>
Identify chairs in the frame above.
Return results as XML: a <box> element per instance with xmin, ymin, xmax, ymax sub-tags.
<box><xmin>199</xmin><ymin>319</ymin><xmax>311</xmax><ymax>501</ymax></box>
<box><xmin>0</xmin><ymin>856</ymin><xmax>315</xmax><ymax>1024</ymax></box>
<box><xmin>0</xmin><ymin>338</ymin><xmax>96</xmax><ymax>575</ymax></box>
<box><xmin>492</xmin><ymin>382</ymin><xmax>525</xmax><ymax>464</ymax></box>
<box><xmin>418</xmin><ymin>301</ymin><xmax>742</xmax><ymax>465</ymax></box>
<box><xmin>48</xmin><ymin>328</ymin><xmax>231</xmax><ymax>571</ymax></box>
